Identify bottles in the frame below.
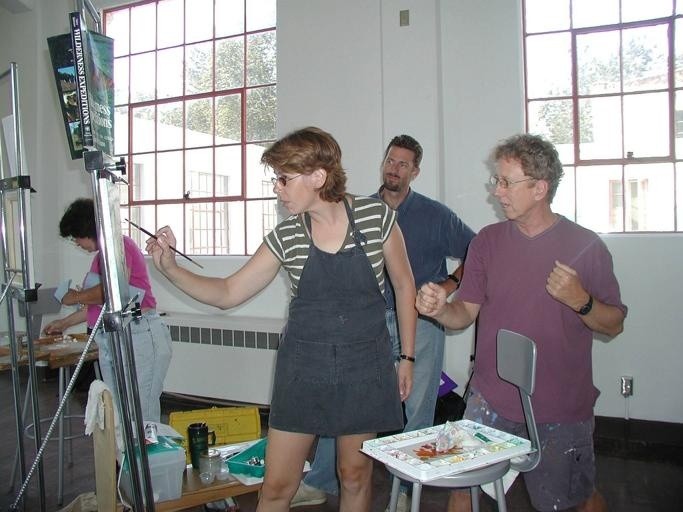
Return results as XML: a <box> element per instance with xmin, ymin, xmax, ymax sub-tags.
<box><xmin>199</xmin><ymin>449</ymin><xmax>222</xmax><ymax>473</ymax></box>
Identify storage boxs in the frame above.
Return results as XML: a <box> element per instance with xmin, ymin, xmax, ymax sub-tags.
<box><xmin>119</xmin><ymin>434</ymin><xmax>186</xmax><ymax>504</ymax></box>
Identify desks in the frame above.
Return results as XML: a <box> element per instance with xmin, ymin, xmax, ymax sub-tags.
<box><xmin>146</xmin><ymin>459</ymin><xmax>263</xmax><ymax>512</ymax></box>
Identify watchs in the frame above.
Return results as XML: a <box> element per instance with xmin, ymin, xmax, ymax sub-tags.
<box><xmin>572</xmin><ymin>293</ymin><xmax>594</xmax><ymax>316</ymax></box>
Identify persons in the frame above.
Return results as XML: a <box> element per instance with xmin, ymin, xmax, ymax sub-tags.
<box><xmin>414</xmin><ymin>132</ymin><xmax>630</xmax><ymax>512</ymax></box>
<box><xmin>42</xmin><ymin>196</ymin><xmax>173</xmax><ymax>469</ymax></box>
<box><xmin>288</xmin><ymin>133</ymin><xmax>476</xmax><ymax>512</ymax></box>
<box><xmin>143</xmin><ymin>124</ymin><xmax>416</xmax><ymax>511</ymax></box>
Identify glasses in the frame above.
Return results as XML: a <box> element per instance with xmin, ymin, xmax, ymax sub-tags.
<box><xmin>70</xmin><ymin>236</ymin><xmax>79</xmax><ymax>243</ymax></box>
<box><xmin>270</xmin><ymin>175</ymin><xmax>301</xmax><ymax>187</ymax></box>
<box><xmin>490</xmin><ymin>176</ymin><xmax>539</xmax><ymax>188</ymax></box>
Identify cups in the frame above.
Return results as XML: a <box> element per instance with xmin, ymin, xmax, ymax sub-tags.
<box><xmin>190</xmin><ymin>453</ymin><xmax>208</xmax><ymax>468</ymax></box>
<box><xmin>187</xmin><ymin>422</ymin><xmax>216</xmax><ymax>452</ymax></box>
<box><xmin>216</xmin><ymin>464</ymin><xmax>230</xmax><ymax>481</ymax></box>
<box><xmin>199</xmin><ymin>472</ymin><xmax>215</xmax><ymax>485</ymax></box>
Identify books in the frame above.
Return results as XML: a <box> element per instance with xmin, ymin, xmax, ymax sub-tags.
<box><xmin>42</xmin><ymin>12</ymin><xmax>115</xmax><ymax>158</ymax></box>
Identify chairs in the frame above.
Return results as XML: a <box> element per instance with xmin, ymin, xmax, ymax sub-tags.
<box><xmin>382</xmin><ymin>329</ymin><xmax>543</xmax><ymax>511</ymax></box>
<box><xmin>9</xmin><ymin>287</ymin><xmax>98</xmax><ymax>508</ymax></box>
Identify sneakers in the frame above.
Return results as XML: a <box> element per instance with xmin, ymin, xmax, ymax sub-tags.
<box><xmin>384</xmin><ymin>491</ymin><xmax>411</xmax><ymax>511</ymax></box>
<box><xmin>289</xmin><ymin>480</ymin><xmax>326</xmax><ymax>507</ymax></box>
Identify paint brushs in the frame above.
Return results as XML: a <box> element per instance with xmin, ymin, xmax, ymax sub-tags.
<box><xmin>124</xmin><ymin>219</ymin><xmax>204</xmax><ymax>268</ymax></box>
<box><xmin>120</xmin><ymin>292</ymin><xmax>140</xmax><ymax>311</ymax></box>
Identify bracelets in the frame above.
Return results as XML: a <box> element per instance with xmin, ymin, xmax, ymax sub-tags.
<box><xmin>399</xmin><ymin>354</ymin><xmax>416</xmax><ymax>362</ymax></box>
<box><xmin>448</xmin><ymin>274</ymin><xmax>461</xmax><ymax>290</ymax></box>
<box><xmin>76</xmin><ymin>293</ymin><xmax>89</xmax><ymax>309</ymax></box>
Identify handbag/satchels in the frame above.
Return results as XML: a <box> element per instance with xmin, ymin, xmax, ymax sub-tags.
<box><xmin>81</xmin><ymin>272</ymin><xmax>145</xmax><ymax>315</ymax></box>
